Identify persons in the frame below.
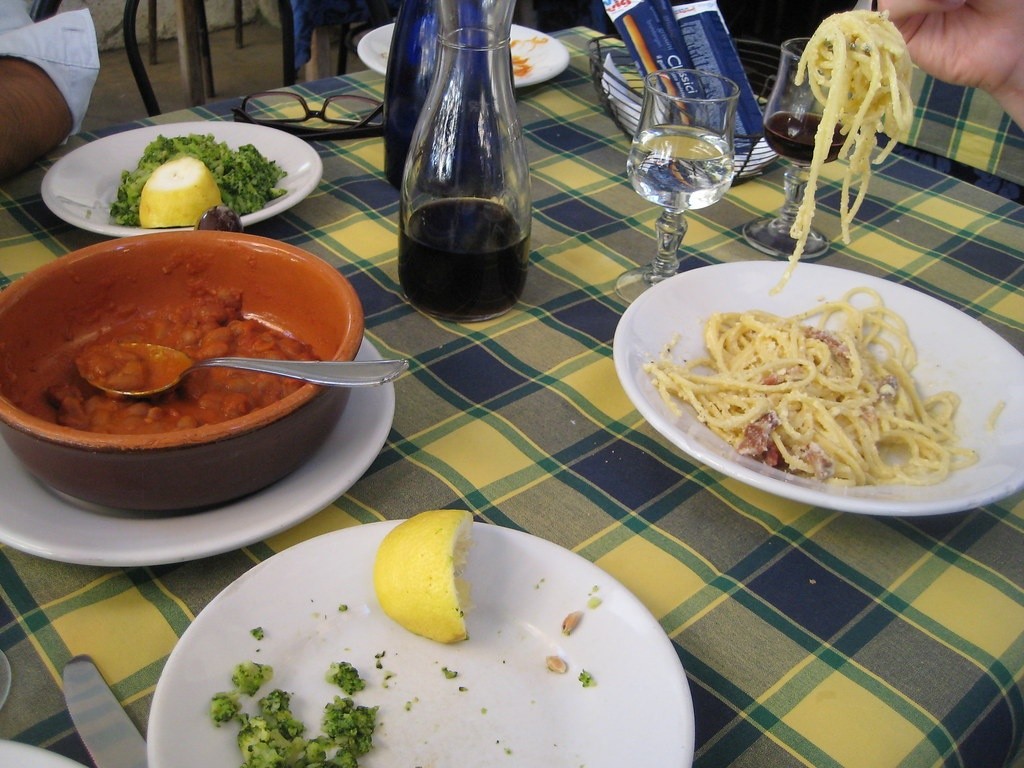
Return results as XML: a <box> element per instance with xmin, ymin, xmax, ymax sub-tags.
<box><xmin>0</xmin><ymin>0</ymin><xmax>101</xmax><ymax>183</ymax></box>
<box><xmin>869</xmin><ymin>0</ymin><xmax>1024</xmax><ymax>130</ymax></box>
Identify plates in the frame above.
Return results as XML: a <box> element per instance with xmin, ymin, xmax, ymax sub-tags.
<box><xmin>0</xmin><ymin>739</ymin><xmax>90</xmax><ymax>768</ymax></box>
<box><xmin>147</xmin><ymin>519</ymin><xmax>695</xmax><ymax>768</ymax></box>
<box><xmin>41</xmin><ymin>121</ymin><xmax>324</xmax><ymax>237</ymax></box>
<box><xmin>613</xmin><ymin>260</ymin><xmax>1024</xmax><ymax>516</ymax></box>
<box><xmin>358</xmin><ymin>23</ymin><xmax>570</xmax><ymax>88</ymax></box>
<box><xmin>0</xmin><ymin>337</ymin><xmax>395</xmax><ymax>567</ymax></box>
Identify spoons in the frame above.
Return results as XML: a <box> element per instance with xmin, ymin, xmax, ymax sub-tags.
<box><xmin>76</xmin><ymin>344</ymin><xmax>410</xmax><ymax>397</ymax></box>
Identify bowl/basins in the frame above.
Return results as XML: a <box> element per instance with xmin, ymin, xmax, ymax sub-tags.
<box><xmin>0</xmin><ymin>230</ymin><xmax>364</xmax><ymax>512</ymax></box>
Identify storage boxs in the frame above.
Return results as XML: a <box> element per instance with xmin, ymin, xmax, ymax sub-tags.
<box><xmin>602</xmin><ymin>0</ymin><xmax>764</xmax><ymax>153</ymax></box>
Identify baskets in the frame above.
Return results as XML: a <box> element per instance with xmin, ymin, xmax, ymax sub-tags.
<box><xmin>586</xmin><ymin>33</ymin><xmax>817</xmax><ymax>187</ymax></box>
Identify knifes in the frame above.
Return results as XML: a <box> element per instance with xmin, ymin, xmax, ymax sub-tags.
<box><xmin>63</xmin><ymin>655</ymin><xmax>147</xmax><ymax>768</ymax></box>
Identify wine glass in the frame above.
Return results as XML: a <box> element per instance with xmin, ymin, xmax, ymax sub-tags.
<box><xmin>744</xmin><ymin>37</ymin><xmax>850</xmax><ymax>259</ymax></box>
<box><xmin>615</xmin><ymin>70</ymin><xmax>741</xmax><ymax>304</ymax></box>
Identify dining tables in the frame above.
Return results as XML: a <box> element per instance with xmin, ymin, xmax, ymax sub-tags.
<box><xmin>1</xmin><ymin>28</ymin><xmax>1024</xmax><ymax>767</ymax></box>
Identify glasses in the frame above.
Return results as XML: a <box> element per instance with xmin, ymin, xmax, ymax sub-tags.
<box><xmin>232</xmin><ymin>91</ymin><xmax>386</xmax><ymax>139</ymax></box>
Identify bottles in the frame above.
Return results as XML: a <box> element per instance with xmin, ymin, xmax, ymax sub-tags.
<box><xmin>384</xmin><ymin>0</ymin><xmax>441</xmax><ymax>192</ymax></box>
<box><xmin>398</xmin><ymin>0</ymin><xmax>532</xmax><ymax>322</ymax></box>
